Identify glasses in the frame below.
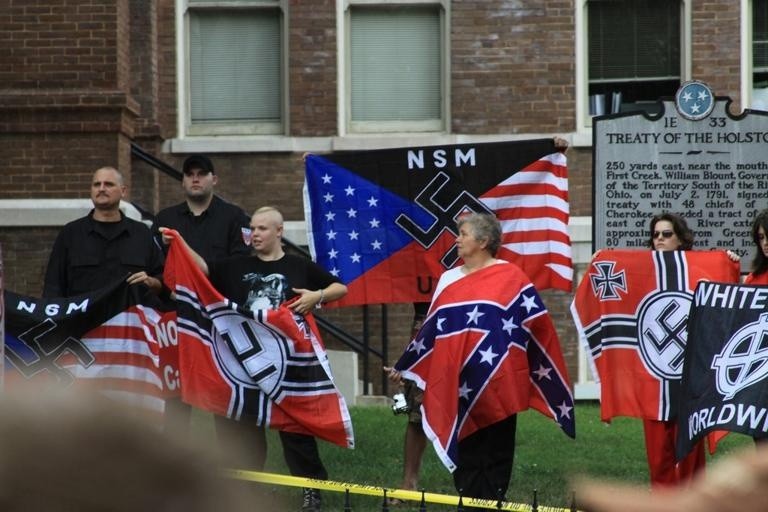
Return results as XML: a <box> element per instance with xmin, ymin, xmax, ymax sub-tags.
<box><xmin>653</xmin><ymin>230</ymin><xmax>676</xmax><ymax>238</ymax></box>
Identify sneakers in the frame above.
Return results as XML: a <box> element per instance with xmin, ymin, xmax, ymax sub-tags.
<box><xmin>302</xmin><ymin>487</ymin><xmax>321</xmax><ymax>510</ymax></box>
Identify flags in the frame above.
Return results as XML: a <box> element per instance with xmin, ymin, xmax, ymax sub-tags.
<box><xmin>302</xmin><ymin>137</ymin><xmax>572</xmax><ymax>309</ymax></box>
<box><xmin>568</xmin><ymin>249</ymin><xmax>742</xmax><ymax>455</ymax></box>
<box><xmin>0</xmin><ymin>272</ymin><xmax>168</xmax><ymax>469</ymax></box>
<box><xmin>161</xmin><ymin>227</ymin><xmax>355</xmax><ymax>450</ymax></box>
<box><xmin>388</xmin><ymin>260</ymin><xmax>576</xmax><ymax>474</ymax></box>
<box><xmin>674</xmin><ymin>281</ymin><xmax>768</xmax><ymax>465</ymax></box>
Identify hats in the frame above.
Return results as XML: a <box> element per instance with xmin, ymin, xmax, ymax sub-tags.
<box><xmin>182</xmin><ymin>155</ymin><xmax>215</xmax><ymax>174</ymax></box>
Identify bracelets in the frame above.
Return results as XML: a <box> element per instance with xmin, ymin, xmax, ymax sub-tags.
<box><xmin>316</xmin><ymin>288</ymin><xmax>323</xmax><ymax>310</ymax></box>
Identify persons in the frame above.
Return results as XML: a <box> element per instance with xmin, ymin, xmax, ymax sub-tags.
<box><xmin>698</xmin><ymin>209</ymin><xmax>767</xmax><ymax>460</ymax></box>
<box><xmin>41</xmin><ymin>167</ymin><xmax>170</xmax><ymax>297</ymax></box>
<box><xmin>301</xmin><ymin>138</ymin><xmax>572</xmax><ymax>506</ymax></box>
<box><xmin>157</xmin><ymin>206</ymin><xmax>350</xmax><ymax>512</ymax></box>
<box><xmin>381</xmin><ymin>213</ymin><xmax>529</xmax><ymax>511</ymax></box>
<box><xmin>151</xmin><ymin>154</ymin><xmax>248</xmax><ymax>470</ymax></box>
<box><xmin>591</xmin><ymin>213</ymin><xmax>742</xmax><ymax>498</ymax></box>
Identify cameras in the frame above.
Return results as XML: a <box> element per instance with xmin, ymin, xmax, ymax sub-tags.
<box><xmin>391</xmin><ymin>391</ymin><xmax>409</xmax><ymax>415</ymax></box>
